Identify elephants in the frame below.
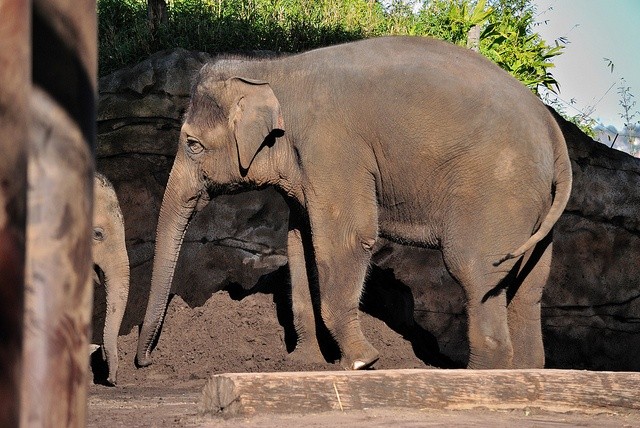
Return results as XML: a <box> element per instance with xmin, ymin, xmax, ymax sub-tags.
<box><xmin>92</xmin><ymin>171</ymin><xmax>131</xmax><ymax>384</ymax></box>
<box><xmin>136</xmin><ymin>34</ymin><xmax>574</xmax><ymax>368</ymax></box>
